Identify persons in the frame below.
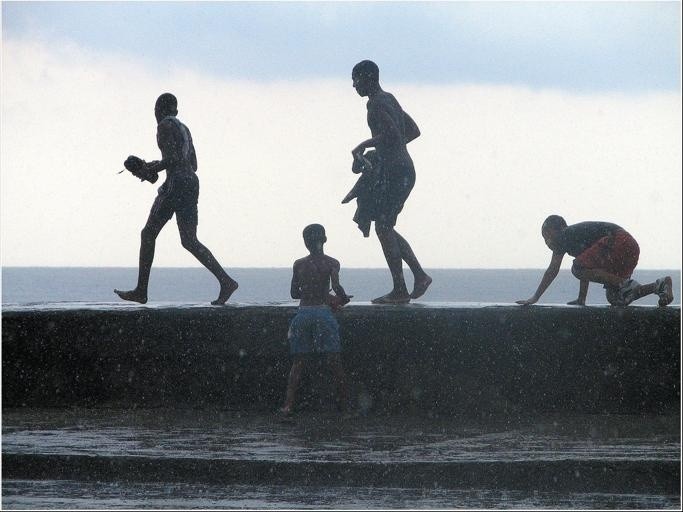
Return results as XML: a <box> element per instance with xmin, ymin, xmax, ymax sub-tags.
<box><xmin>351</xmin><ymin>60</ymin><xmax>432</xmax><ymax>303</ymax></box>
<box><xmin>281</xmin><ymin>224</ymin><xmax>360</xmax><ymax>420</ymax></box>
<box><xmin>516</xmin><ymin>215</ymin><xmax>674</xmax><ymax>307</ymax></box>
<box><xmin>114</xmin><ymin>93</ymin><xmax>238</xmax><ymax>305</ymax></box>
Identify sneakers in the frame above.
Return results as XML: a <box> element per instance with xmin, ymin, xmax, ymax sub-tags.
<box><xmin>618</xmin><ymin>280</ymin><xmax>638</xmax><ymax>304</ymax></box>
<box><xmin>655</xmin><ymin>276</ymin><xmax>673</xmax><ymax>306</ymax></box>
